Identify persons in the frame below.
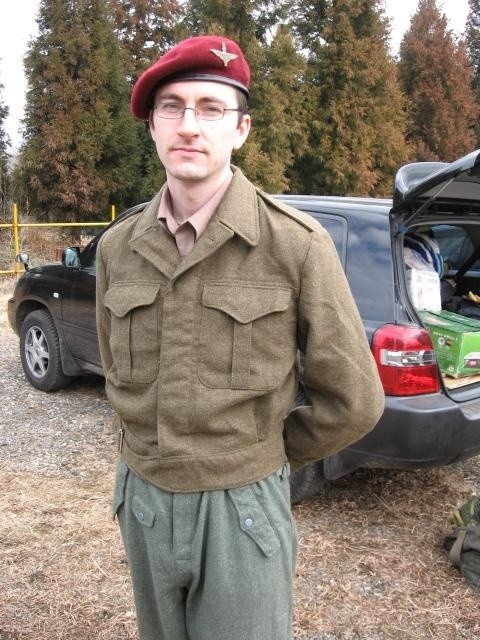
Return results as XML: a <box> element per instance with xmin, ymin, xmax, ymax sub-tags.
<box><xmin>95</xmin><ymin>36</ymin><xmax>386</xmax><ymax>640</ymax></box>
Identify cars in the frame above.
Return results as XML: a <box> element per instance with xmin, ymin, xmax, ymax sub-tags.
<box><xmin>6</xmin><ymin>149</ymin><xmax>479</xmax><ymax>507</ymax></box>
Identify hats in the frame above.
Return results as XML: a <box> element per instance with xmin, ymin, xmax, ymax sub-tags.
<box><xmin>131</xmin><ymin>35</ymin><xmax>250</xmax><ymax>121</ymax></box>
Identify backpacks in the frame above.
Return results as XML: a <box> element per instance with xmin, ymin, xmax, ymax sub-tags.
<box><xmin>435</xmin><ymin>495</ymin><xmax>480</xmax><ymax>593</ymax></box>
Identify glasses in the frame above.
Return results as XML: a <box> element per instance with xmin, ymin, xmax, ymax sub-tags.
<box><xmin>155</xmin><ymin>100</ymin><xmax>240</xmax><ymax>121</ymax></box>
